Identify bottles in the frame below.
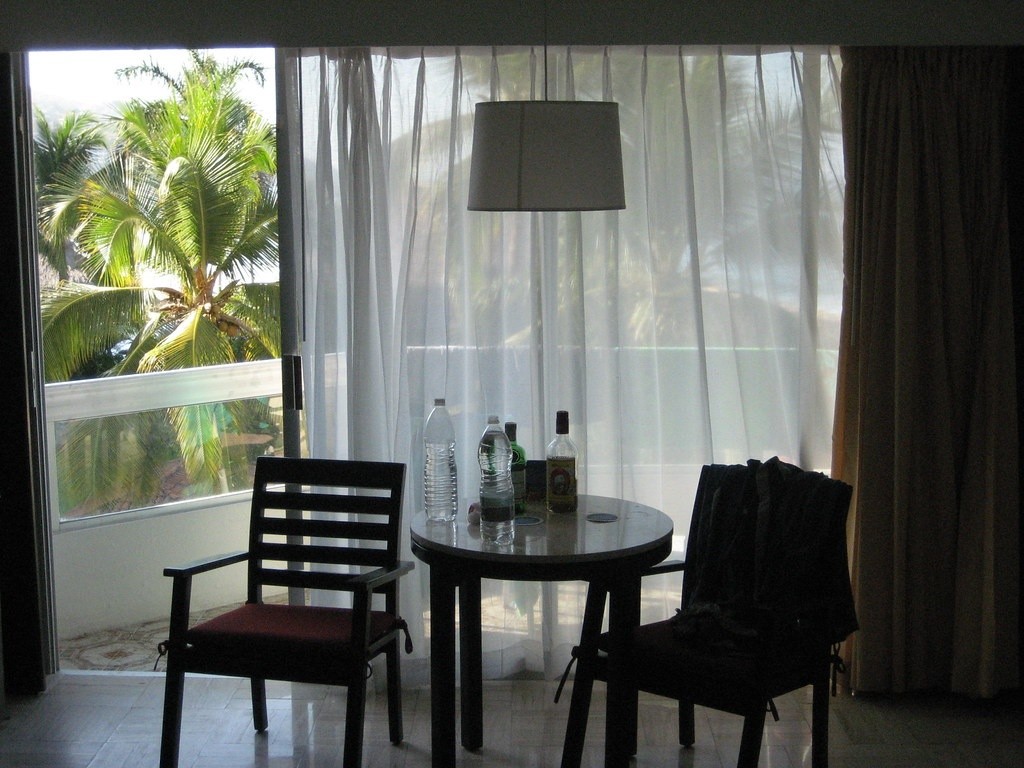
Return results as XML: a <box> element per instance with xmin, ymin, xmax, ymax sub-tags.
<box><xmin>477</xmin><ymin>416</ymin><xmax>516</xmax><ymax>546</ymax></box>
<box><xmin>504</xmin><ymin>422</ymin><xmax>526</xmax><ymax>511</ymax></box>
<box><xmin>422</xmin><ymin>398</ymin><xmax>458</xmax><ymax>522</ymax></box>
<box><xmin>546</xmin><ymin>411</ymin><xmax>579</xmax><ymax>513</ymax></box>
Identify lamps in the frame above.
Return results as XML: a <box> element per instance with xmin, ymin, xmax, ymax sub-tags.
<box><xmin>466</xmin><ymin>1</ymin><xmax>628</xmax><ymax>213</ymax></box>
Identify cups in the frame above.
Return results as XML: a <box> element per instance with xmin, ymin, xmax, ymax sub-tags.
<box><xmin>526</xmin><ymin>460</ymin><xmax>546</xmax><ymax>498</ymax></box>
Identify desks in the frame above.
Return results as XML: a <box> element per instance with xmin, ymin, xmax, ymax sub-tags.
<box><xmin>408</xmin><ymin>495</ymin><xmax>674</xmax><ymax>768</ymax></box>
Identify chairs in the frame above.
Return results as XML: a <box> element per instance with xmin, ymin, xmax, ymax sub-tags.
<box><xmin>560</xmin><ymin>460</ymin><xmax>863</xmax><ymax>768</ymax></box>
<box><xmin>156</xmin><ymin>455</ymin><xmax>415</xmax><ymax>768</ymax></box>
